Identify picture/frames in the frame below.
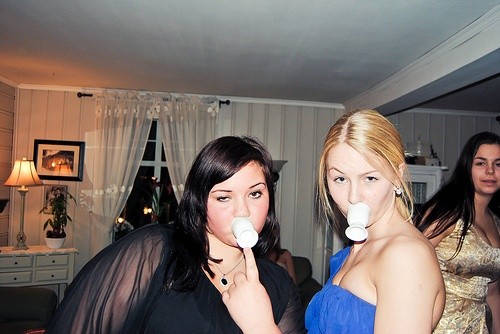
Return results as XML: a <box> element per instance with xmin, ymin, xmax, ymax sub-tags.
<box><xmin>33</xmin><ymin>138</ymin><xmax>84</xmax><ymax>180</ymax></box>
<box><xmin>44</xmin><ymin>185</ymin><xmax>68</xmax><ymax>216</ymax></box>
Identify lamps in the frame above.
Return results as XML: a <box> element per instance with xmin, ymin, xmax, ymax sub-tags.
<box><xmin>4</xmin><ymin>158</ymin><xmax>45</xmax><ymax>253</ymax></box>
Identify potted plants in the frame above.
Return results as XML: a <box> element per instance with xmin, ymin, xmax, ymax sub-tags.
<box><xmin>40</xmin><ymin>194</ymin><xmax>78</xmax><ymax>248</ymax></box>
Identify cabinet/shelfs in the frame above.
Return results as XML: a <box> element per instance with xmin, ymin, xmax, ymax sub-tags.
<box><xmin>0</xmin><ymin>245</ymin><xmax>77</xmax><ymax>308</ymax></box>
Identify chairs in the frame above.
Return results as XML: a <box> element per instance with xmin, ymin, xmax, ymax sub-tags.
<box><xmin>0</xmin><ymin>286</ymin><xmax>58</xmax><ymax>334</ymax></box>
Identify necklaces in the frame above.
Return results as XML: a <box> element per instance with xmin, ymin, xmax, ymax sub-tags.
<box><xmin>215</xmin><ymin>254</ymin><xmax>244</xmax><ymax>285</ymax></box>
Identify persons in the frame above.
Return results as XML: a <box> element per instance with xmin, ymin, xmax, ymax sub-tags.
<box><xmin>303</xmin><ymin>107</ymin><xmax>446</xmax><ymax>334</ymax></box>
<box><xmin>411</xmin><ymin>131</ymin><xmax>500</xmax><ymax>334</ymax></box>
<box><xmin>42</xmin><ymin>136</ymin><xmax>307</xmax><ymax>334</ymax></box>
<box><xmin>265</xmin><ymin>238</ymin><xmax>297</xmax><ymax>287</ymax></box>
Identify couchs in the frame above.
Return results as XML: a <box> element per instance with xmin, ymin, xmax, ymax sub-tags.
<box><xmin>293</xmin><ymin>256</ymin><xmax>323</xmax><ymax>308</ymax></box>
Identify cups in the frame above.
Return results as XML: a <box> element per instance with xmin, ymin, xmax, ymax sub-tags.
<box><xmin>345</xmin><ymin>203</ymin><xmax>370</xmax><ymax>242</ymax></box>
<box><xmin>231</xmin><ymin>217</ymin><xmax>258</xmax><ymax>249</ymax></box>
